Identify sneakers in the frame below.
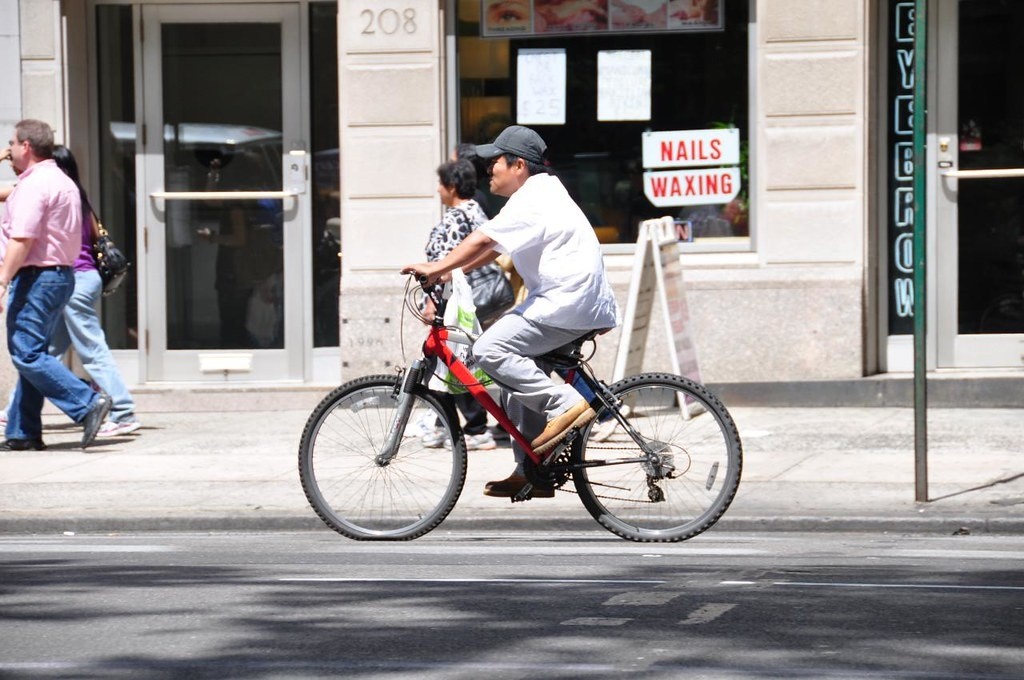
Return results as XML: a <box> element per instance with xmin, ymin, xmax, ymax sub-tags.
<box><xmin>484</xmin><ymin>471</ymin><xmax>553</xmax><ymax>497</ymax></box>
<box><xmin>424</xmin><ymin>427</ymin><xmax>449</xmax><ymax>447</ymax></box>
<box><xmin>97</xmin><ymin>421</ymin><xmax>139</xmax><ymax>437</ymax></box>
<box><xmin>0</xmin><ymin>412</ymin><xmax>8</xmax><ymax>426</ymax></box>
<box><xmin>443</xmin><ymin>430</ymin><xmax>496</xmax><ymax>450</ymax></box>
<box><xmin>531</xmin><ymin>400</ymin><xmax>595</xmax><ymax>453</ymax></box>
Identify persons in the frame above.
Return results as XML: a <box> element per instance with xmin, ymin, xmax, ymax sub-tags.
<box><xmin>399</xmin><ymin>125</ymin><xmax>616</xmax><ymax>498</ymax></box>
<box><xmin>403</xmin><ymin>143</ymin><xmax>630</xmax><ymax>452</ymax></box>
<box><xmin>0</xmin><ymin>119</ymin><xmax>140</xmax><ymax>451</ymax></box>
<box><xmin>184</xmin><ymin>149</ymin><xmax>247</xmax><ymax>346</ymax></box>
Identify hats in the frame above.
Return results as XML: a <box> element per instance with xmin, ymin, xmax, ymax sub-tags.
<box><xmin>475</xmin><ymin>125</ymin><xmax>551</xmax><ymax>167</ymax></box>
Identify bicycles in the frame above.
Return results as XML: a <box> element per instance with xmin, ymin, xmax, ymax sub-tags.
<box><xmin>298</xmin><ymin>269</ymin><xmax>744</xmax><ymax>544</ymax></box>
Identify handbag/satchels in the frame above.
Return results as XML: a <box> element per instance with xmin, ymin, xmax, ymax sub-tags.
<box><xmin>86</xmin><ymin>199</ymin><xmax>126</xmax><ymax>296</ymax></box>
<box><xmin>421</xmin><ymin>266</ymin><xmax>496</xmax><ymax>394</ymax></box>
<box><xmin>462</xmin><ymin>264</ymin><xmax>514</xmax><ymax>320</ymax></box>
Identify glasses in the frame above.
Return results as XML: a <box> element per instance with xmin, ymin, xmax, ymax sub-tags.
<box><xmin>9</xmin><ymin>141</ymin><xmax>22</xmax><ymax>146</ymax></box>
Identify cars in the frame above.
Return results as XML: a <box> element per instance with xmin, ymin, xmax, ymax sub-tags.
<box><xmin>105</xmin><ymin>118</ymin><xmax>341</xmax><ymax>351</ymax></box>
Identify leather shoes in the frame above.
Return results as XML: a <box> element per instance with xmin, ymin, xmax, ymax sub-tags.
<box><xmin>0</xmin><ymin>439</ymin><xmax>46</xmax><ymax>451</ymax></box>
<box><xmin>82</xmin><ymin>398</ymin><xmax>109</xmax><ymax>448</ymax></box>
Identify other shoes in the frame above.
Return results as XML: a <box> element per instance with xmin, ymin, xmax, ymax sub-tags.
<box><xmin>401</xmin><ymin>420</ymin><xmax>435</xmax><ymax>437</ymax></box>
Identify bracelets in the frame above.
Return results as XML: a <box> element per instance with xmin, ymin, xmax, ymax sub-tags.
<box><xmin>0</xmin><ymin>282</ymin><xmax>8</xmax><ymax>289</ymax></box>
<box><xmin>209</xmin><ymin>231</ymin><xmax>216</xmax><ymax>243</ymax></box>
